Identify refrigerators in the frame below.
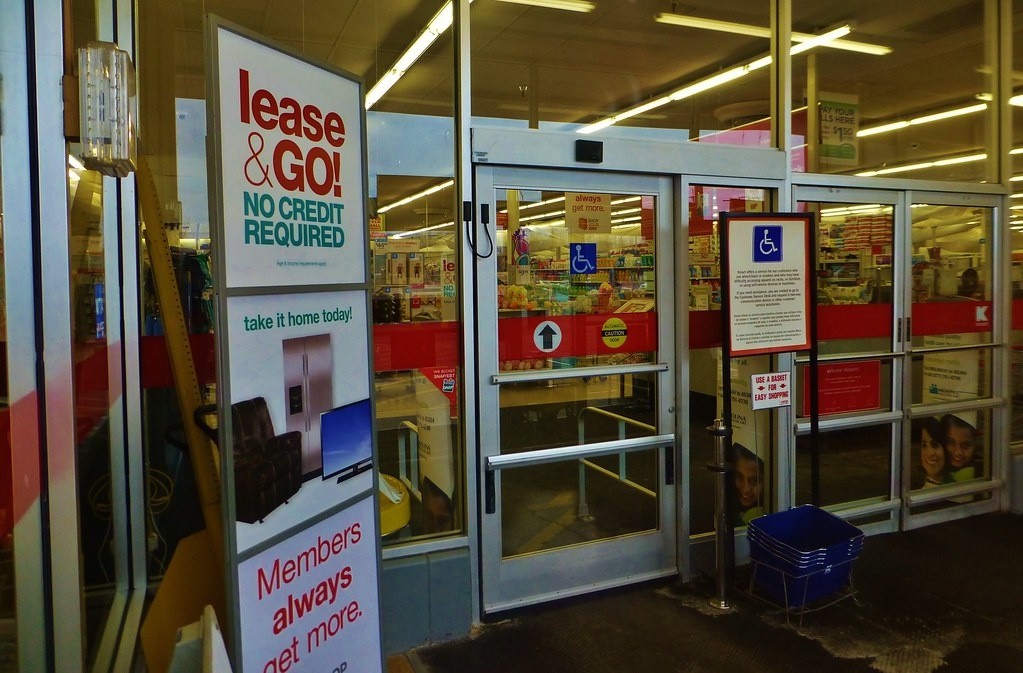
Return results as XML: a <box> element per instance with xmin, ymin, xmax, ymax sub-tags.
<box><xmin>281</xmin><ymin>333</ymin><xmax>334</xmax><ymax>482</ymax></box>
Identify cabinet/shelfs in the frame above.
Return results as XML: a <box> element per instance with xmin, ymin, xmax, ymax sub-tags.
<box><xmin>689</xmin><ymin>262</ymin><xmax>720</xmax><ymax>294</ymax></box>
<box><xmin>819</xmin><ymin>248</ymin><xmax>871</xmax><ymax>282</ymax></box>
<box><xmin>530</xmin><ymin>266</ymin><xmax>654</xmax><ymax>302</ymax></box>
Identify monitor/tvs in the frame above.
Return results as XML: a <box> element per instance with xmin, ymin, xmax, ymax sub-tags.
<box><xmin>320</xmin><ymin>398</ymin><xmax>374</xmax><ymax>483</ymax></box>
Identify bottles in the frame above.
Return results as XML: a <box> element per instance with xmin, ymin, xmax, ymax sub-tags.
<box><xmin>533</xmin><ymin>249</ymin><xmax>655</xmax><ymax>316</ymax></box>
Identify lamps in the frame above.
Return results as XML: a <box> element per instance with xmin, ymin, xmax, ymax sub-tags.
<box><xmin>853</xmin><ymin>87</ymin><xmax>1023</xmax><ymax>177</ymax></box>
<box><xmin>578</xmin><ymin>0</ymin><xmax>895</xmax><ymax>134</ymax></box>
<box><xmin>365</xmin><ymin>0</ymin><xmax>596</xmax><ymax>111</ymax></box>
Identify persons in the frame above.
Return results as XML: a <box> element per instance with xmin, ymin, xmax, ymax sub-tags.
<box><xmin>958</xmin><ymin>268</ymin><xmax>985</xmax><ymax>301</ymax></box>
<box><xmin>838</xmin><ymin>254</ymin><xmax>862</xmax><ymax>286</ymax></box>
<box><xmin>731</xmin><ymin>443</ymin><xmax>764</xmax><ymax>528</ymax></box>
<box><xmin>920</xmin><ymin>412</ymin><xmax>977</xmax><ymax>490</ymax></box>
